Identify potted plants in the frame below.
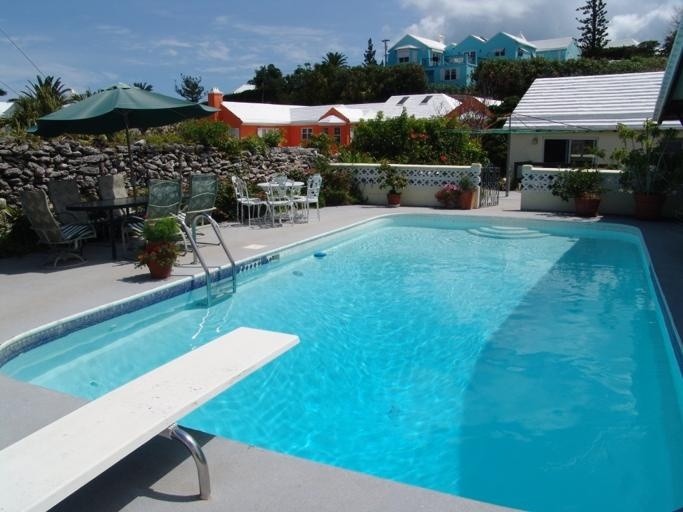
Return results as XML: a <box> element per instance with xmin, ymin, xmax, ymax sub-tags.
<box><xmin>131</xmin><ymin>214</ymin><xmax>186</xmax><ymax>278</ymax></box>
<box><xmin>546</xmin><ymin>155</ymin><xmax>605</xmax><ymax>219</ymax></box>
<box><xmin>608</xmin><ymin>116</ymin><xmax>683</xmax><ymax>221</ymax></box>
<box><xmin>375</xmin><ymin>156</ymin><xmax>412</xmax><ymax>207</ymax></box>
<box><xmin>455</xmin><ymin>170</ymin><xmax>480</xmax><ymax>209</ymax></box>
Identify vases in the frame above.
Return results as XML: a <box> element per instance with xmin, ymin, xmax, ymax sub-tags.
<box><xmin>440</xmin><ymin>198</ymin><xmax>457</xmax><ymax>209</ymax></box>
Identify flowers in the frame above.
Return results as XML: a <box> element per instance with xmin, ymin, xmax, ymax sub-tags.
<box><xmin>435</xmin><ymin>183</ymin><xmax>458</xmax><ymax>197</ymax></box>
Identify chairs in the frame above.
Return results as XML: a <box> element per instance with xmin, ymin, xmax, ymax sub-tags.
<box><xmin>19</xmin><ymin>164</ymin><xmax>323</xmax><ymax>263</ymax></box>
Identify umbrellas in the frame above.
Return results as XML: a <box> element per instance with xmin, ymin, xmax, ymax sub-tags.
<box><xmin>25</xmin><ymin>79</ymin><xmax>221</xmax><ymax>204</ymax></box>
